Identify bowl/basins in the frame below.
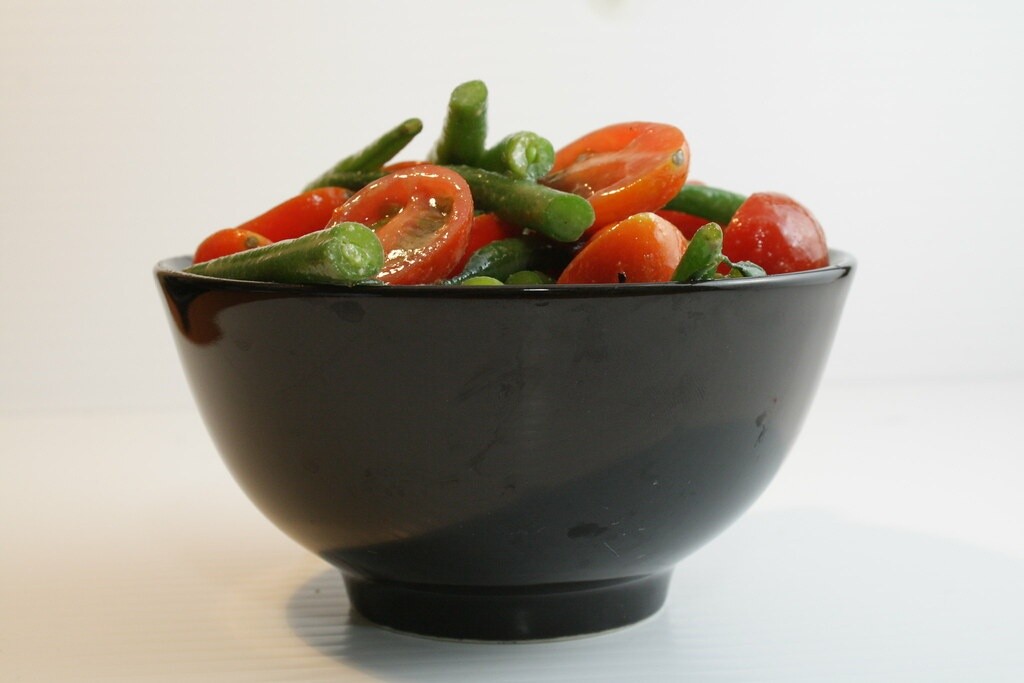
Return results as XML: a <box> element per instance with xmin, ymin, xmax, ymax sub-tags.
<box><xmin>154</xmin><ymin>250</ymin><xmax>859</xmax><ymax>641</ymax></box>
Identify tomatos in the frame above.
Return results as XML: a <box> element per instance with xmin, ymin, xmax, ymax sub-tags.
<box><xmin>193</xmin><ymin>118</ymin><xmax>828</xmax><ymax>286</ymax></box>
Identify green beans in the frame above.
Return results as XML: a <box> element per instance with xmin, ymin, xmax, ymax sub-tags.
<box><xmin>179</xmin><ymin>80</ymin><xmax>770</xmax><ymax>286</ymax></box>
